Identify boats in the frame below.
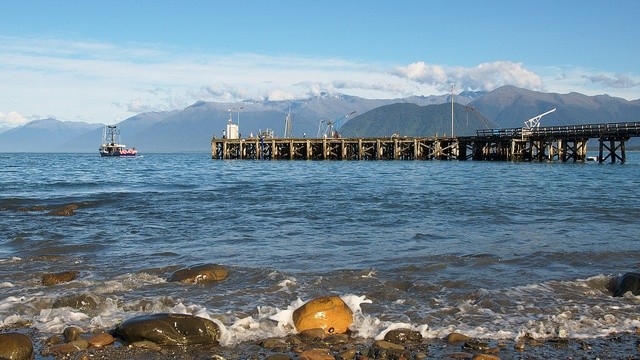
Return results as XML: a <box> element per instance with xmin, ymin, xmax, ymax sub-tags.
<box><xmin>587</xmin><ymin>156</ymin><xmax>604</xmax><ymax>162</ymax></box>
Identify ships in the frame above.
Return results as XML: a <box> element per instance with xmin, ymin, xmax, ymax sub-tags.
<box><xmin>99</xmin><ymin>125</ymin><xmax>137</xmax><ymax>156</ymax></box>
